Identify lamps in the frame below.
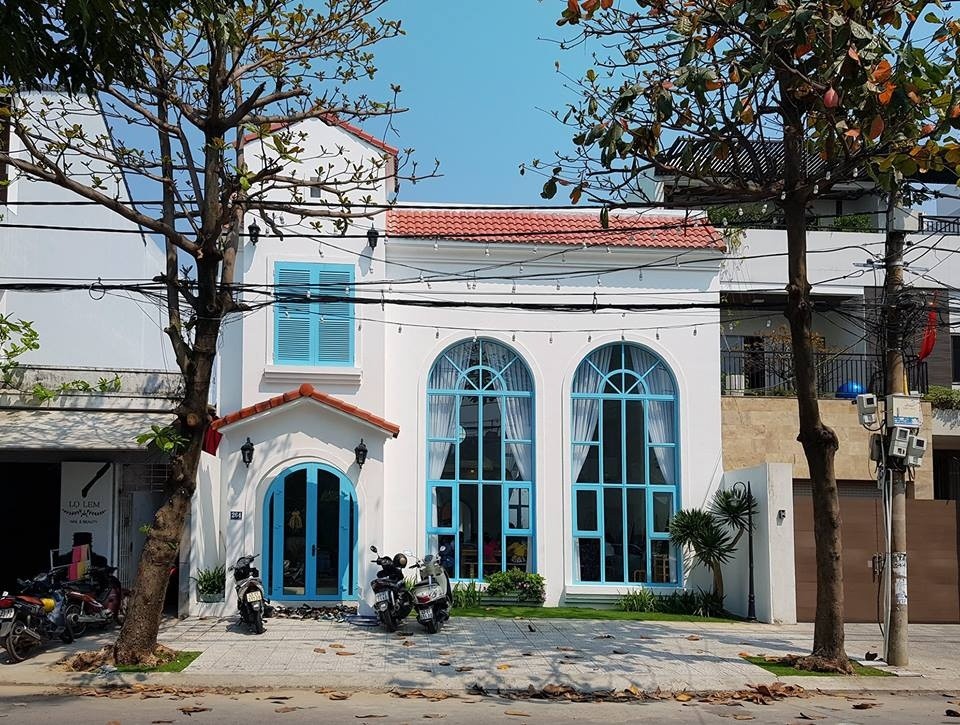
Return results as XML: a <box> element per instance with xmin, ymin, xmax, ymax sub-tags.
<box><xmin>354</xmin><ymin>437</ymin><xmax>369</xmax><ymax>469</ymax></box>
<box><xmin>367</xmin><ymin>223</ymin><xmax>379</xmax><ymax>251</ymax></box>
<box><xmin>248</xmin><ymin>218</ymin><xmax>261</xmax><ymax>246</ymax></box>
<box><xmin>240</xmin><ymin>436</ymin><xmax>256</xmax><ymax>468</ymax></box>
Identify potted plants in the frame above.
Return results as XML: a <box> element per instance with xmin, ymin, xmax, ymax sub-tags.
<box><xmin>188</xmin><ymin>563</ymin><xmax>228</xmax><ymax>602</ymax></box>
<box><xmin>833</xmin><ymin>214</ymin><xmax>856</xmax><ymax>232</ymax></box>
<box><xmin>852</xmin><ymin>214</ymin><xmax>873</xmax><ymax>233</ymax></box>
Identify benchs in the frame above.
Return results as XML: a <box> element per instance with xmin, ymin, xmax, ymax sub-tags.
<box><xmin>463</xmin><ymin>561</ymin><xmax>527</xmax><ymax>579</ymax></box>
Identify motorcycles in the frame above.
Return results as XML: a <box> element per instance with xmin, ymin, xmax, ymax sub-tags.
<box><xmin>0</xmin><ymin>566</ymin><xmax>75</xmax><ymax>664</ymax></box>
<box><xmin>227</xmin><ymin>552</ymin><xmax>271</xmax><ymax>635</ymax></box>
<box><xmin>369</xmin><ymin>545</ymin><xmax>414</xmax><ymax>633</ymax></box>
<box><xmin>59</xmin><ymin>558</ymin><xmax>131</xmax><ymax>637</ymax></box>
<box><xmin>403</xmin><ymin>546</ymin><xmax>454</xmax><ymax>633</ymax></box>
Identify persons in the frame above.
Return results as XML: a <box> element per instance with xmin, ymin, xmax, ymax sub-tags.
<box><xmin>483</xmin><ymin>536</ymin><xmax>500</xmax><ymax>574</ymax></box>
<box><xmin>438</xmin><ymin>536</ymin><xmax>454</xmax><ymax>571</ymax></box>
<box><xmin>506</xmin><ymin>537</ymin><xmax>528</xmax><ymax>563</ymax></box>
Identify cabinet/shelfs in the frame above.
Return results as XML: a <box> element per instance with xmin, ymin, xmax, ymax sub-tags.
<box><xmin>459</xmin><ymin>544</ymin><xmax>527</xmax><ymax>579</ymax></box>
<box><xmin>654</xmin><ymin>553</ymin><xmax>669</xmax><ymax>583</ymax></box>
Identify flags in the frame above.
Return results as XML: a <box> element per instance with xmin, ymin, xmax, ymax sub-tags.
<box><xmin>916</xmin><ymin>304</ymin><xmax>936</xmax><ymax>360</ymax></box>
